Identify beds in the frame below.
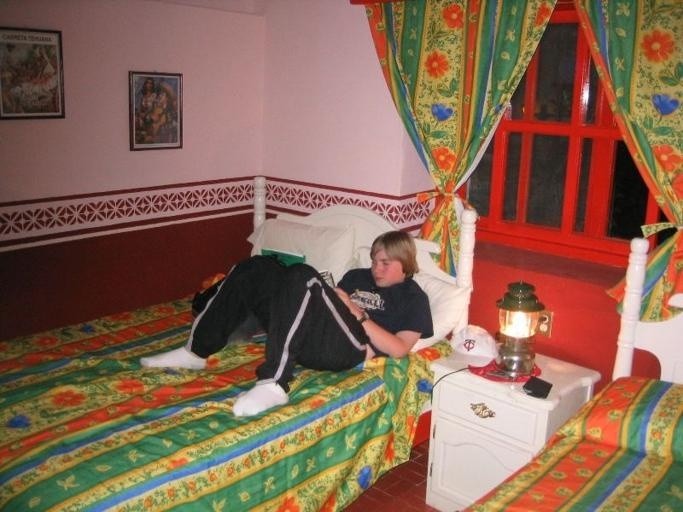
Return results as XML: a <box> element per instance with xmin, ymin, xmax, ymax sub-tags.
<box><xmin>0</xmin><ymin>178</ymin><xmax>477</xmax><ymax>512</ymax></box>
<box><xmin>462</xmin><ymin>240</ymin><xmax>683</xmax><ymax>512</ymax></box>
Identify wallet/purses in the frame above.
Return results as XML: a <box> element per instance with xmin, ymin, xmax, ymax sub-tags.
<box><xmin>524</xmin><ymin>375</ymin><xmax>552</xmax><ymax>398</ymax></box>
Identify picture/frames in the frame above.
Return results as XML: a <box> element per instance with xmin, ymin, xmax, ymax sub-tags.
<box><xmin>128</xmin><ymin>70</ymin><xmax>183</xmax><ymax>150</ymax></box>
<box><xmin>1</xmin><ymin>26</ymin><xmax>66</xmax><ymax>119</ymax></box>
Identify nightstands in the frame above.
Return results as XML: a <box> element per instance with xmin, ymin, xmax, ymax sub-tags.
<box><xmin>425</xmin><ymin>350</ymin><xmax>601</xmax><ymax>512</ymax></box>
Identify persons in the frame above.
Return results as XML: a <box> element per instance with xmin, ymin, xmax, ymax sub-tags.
<box><xmin>134</xmin><ymin>77</ymin><xmax>178</xmax><ymax>145</ymax></box>
<box><xmin>138</xmin><ymin>232</ymin><xmax>437</xmax><ymax>415</ymax></box>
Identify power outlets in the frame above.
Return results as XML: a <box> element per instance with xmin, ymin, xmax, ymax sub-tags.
<box><xmin>539</xmin><ymin>309</ymin><xmax>553</xmax><ymax>337</ymax></box>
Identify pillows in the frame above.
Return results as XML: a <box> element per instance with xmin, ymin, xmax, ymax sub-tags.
<box><xmin>248</xmin><ymin>218</ymin><xmax>469</xmax><ymax>354</ymax></box>
<box><xmin>563</xmin><ymin>374</ymin><xmax>683</xmax><ymax>461</ymax></box>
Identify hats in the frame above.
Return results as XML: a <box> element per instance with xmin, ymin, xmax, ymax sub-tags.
<box><xmin>447</xmin><ymin>324</ymin><xmax>499</xmax><ymax>367</ymax></box>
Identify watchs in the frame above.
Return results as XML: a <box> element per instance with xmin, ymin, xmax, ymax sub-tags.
<box><xmin>356</xmin><ymin>311</ymin><xmax>369</xmax><ymax>325</ymax></box>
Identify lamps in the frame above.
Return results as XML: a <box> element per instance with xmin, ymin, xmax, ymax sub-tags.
<box><xmin>492</xmin><ymin>281</ymin><xmax>544</xmax><ymax>377</ymax></box>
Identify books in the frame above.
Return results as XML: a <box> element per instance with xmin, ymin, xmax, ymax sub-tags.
<box><xmin>318</xmin><ymin>270</ymin><xmax>335</xmax><ymax>291</ymax></box>
<box><xmin>260</xmin><ymin>246</ymin><xmax>306</xmax><ymax>268</ymax></box>
